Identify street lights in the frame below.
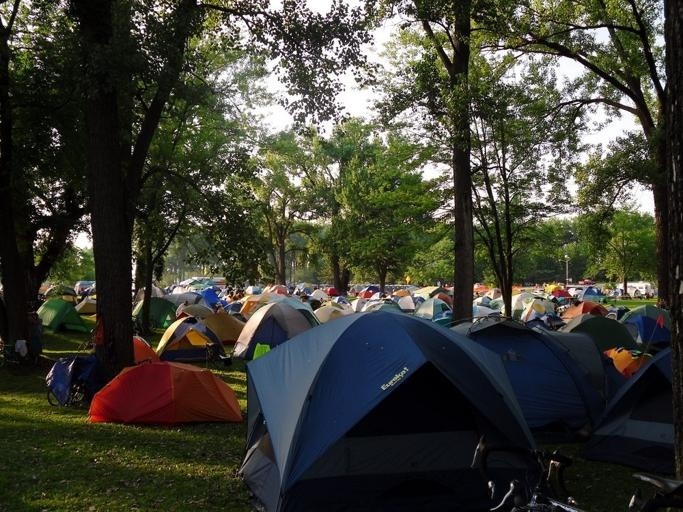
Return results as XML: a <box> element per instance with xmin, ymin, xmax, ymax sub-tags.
<box><xmin>565</xmin><ymin>255</ymin><xmax>571</xmax><ymax>288</ymax></box>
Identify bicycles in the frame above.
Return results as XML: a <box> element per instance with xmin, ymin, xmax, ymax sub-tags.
<box><xmin>46</xmin><ymin>357</ymin><xmax>87</xmax><ymax>406</ymax></box>
<box><xmin>477</xmin><ymin>435</ymin><xmax>682</xmax><ymax>511</ymax></box>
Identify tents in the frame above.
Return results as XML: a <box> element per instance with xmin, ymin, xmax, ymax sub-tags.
<box><xmin>35</xmin><ymin>277</ymin><xmax>676</xmax><ymax>512</ymax></box>
